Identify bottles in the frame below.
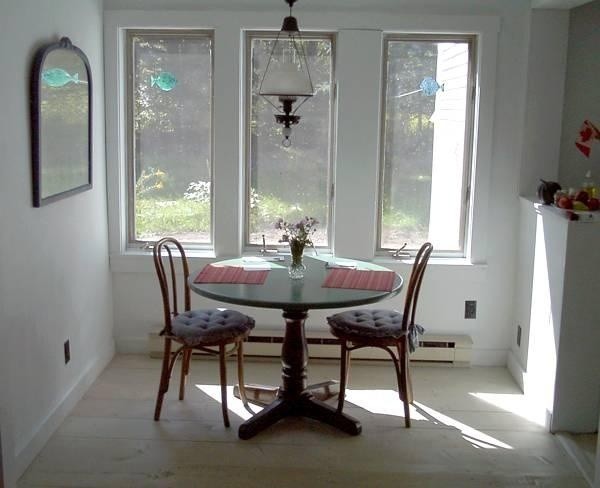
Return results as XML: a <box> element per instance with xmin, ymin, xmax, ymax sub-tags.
<box><xmin>581</xmin><ymin>171</ymin><xmax>594</xmax><ymax>198</ymax></box>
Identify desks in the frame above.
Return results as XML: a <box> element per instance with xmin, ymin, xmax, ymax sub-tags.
<box><xmin>187</xmin><ymin>253</ymin><xmax>399</xmax><ymax>441</ymax></box>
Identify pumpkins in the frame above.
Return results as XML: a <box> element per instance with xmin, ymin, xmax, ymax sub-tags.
<box><xmin>538</xmin><ymin>182</ymin><xmax>561</xmax><ymax>205</ymax></box>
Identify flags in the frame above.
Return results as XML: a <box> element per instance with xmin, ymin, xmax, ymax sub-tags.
<box><xmin>574</xmin><ymin>118</ymin><xmax>600</xmax><ymax>159</ymax></box>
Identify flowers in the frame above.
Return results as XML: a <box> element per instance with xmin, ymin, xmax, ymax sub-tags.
<box><xmin>275</xmin><ymin>217</ymin><xmax>319</xmax><ymax>257</ymax></box>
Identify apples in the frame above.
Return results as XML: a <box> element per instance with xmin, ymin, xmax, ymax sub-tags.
<box><xmin>587</xmin><ymin>198</ymin><xmax>600</xmax><ymax>209</ymax></box>
<box><xmin>558</xmin><ymin>197</ymin><xmax>570</xmax><ymax>208</ymax></box>
<box><xmin>575</xmin><ymin>191</ymin><xmax>589</xmax><ymax>203</ymax></box>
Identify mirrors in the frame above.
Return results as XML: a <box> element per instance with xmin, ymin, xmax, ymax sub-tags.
<box><xmin>30</xmin><ymin>35</ymin><xmax>93</xmax><ymax>208</ymax></box>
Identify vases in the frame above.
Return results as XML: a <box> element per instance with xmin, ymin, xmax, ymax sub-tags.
<box><xmin>286</xmin><ymin>241</ymin><xmax>306</xmax><ymax>279</ymax></box>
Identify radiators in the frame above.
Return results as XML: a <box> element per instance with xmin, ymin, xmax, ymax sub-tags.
<box><xmin>148</xmin><ymin>325</ymin><xmax>474</xmax><ymax>369</ymax></box>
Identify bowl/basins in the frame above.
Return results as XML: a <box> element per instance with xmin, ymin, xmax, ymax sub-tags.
<box><xmin>550</xmin><ymin>201</ymin><xmax>599</xmax><ymax>218</ymax></box>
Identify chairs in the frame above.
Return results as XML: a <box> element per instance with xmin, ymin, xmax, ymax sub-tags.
<box><xmin>153</xmin><ymin>237</ymin><xmax>256</xmax><ymax>429</ymax></box>
<box><xmin>326</xmin><ymin>242</ymin><xmax>433</xmax><ymax>426</ymax></box>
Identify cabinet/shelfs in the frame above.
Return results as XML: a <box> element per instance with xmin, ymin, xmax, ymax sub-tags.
<box><xmin>507</xmin><ymin>197</ymin><xmax>600</xmax><ymax>433</ymax></box>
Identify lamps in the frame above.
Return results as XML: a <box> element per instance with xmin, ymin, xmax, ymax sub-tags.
<box><xmin>257</xmin><ymin>0</ymin><xmax>313</xmax><ymax>148</ymax></box>
